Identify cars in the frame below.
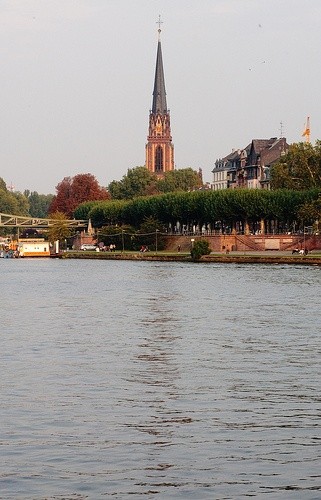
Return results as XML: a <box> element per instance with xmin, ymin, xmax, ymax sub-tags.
<box><xmin>80</xmin><ymin>244</ymin><xmax>98</xmax><ymax>251</ymax></box>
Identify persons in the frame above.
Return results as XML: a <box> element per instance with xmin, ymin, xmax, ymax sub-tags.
<box><xmin>226</xmin><ymin>244</ymin><xmax>230</xmax><ymax>252</ymax></box>
<box><xmin>188</xmin><ymin>223</ymin><xmax>225</xmax><ymax>233</ymax></box>
<box><xmin>95</xmin><ymin>242</ymin><xmax>117</xmax><ymax>253</ymax></box>
<box><xmin>177</xmin><ymin>244</ymin><xmax>181</xmax><ymax>252</ymax></box>
<box><xmin>140</xmin><ymin>245</ymin><xmax>150</xmax><ymax>252</ymax></box>
<box><xmin>279</xmin><ymin>222</ymin><xmax>304</xmax><ymax>232</ymax></box>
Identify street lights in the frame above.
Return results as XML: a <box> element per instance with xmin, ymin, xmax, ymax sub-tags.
<box><xmin>122</xmin><ymin>229</ymin><xmax>125</xmax><ymax>252</ymax></box>
<box><xmin>96</xmin><ymin>230</ymin><xmax>101</xmax><ymax>247</ymax></box>
<box><xmin>155</xmin><ymin>229</ymin><xmax>159</xmax><ymax>254</ymax></box>
<box><xmin>304</xmin><ymin>226</ymin><xmax>308</xmax><ymax>256</ymax></box>
